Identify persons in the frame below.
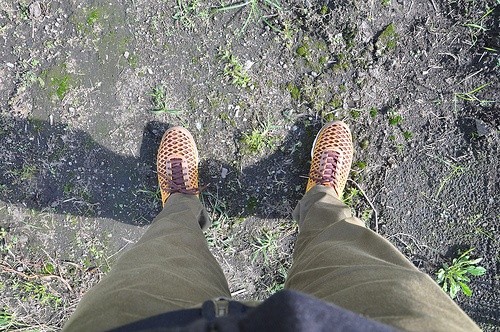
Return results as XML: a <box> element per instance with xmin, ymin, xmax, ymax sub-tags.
<box><xmin>62</xmin><ymin>120</ymin><xmax>485</xmax><ymax>332</ymax></box>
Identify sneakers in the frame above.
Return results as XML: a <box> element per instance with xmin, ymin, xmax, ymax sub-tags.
<box><xmin>305</xmin><ymin>121</ymin><xmax>354</xmax><ymax>196</ymax></box>
<box><xmin>157</xmin><ymin>126</ymin><xmax>199</xmax><ymax>209</ymax></box>
